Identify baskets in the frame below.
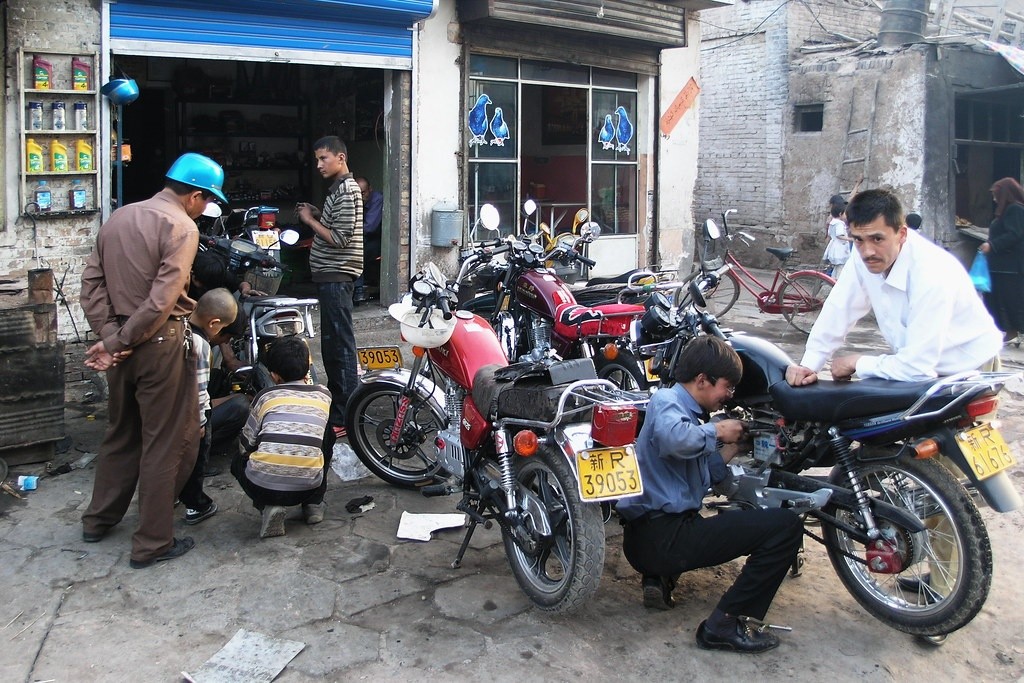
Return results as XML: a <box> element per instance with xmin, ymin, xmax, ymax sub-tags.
<box><xmin>247</xmin><ymin>269</ymin><xmax>284</xmax><ymax>296</ymax></box>
<box><xmin>697</xmin><ymin>234</ymin><xmax>728</xmax><ymax>270</ymax></box>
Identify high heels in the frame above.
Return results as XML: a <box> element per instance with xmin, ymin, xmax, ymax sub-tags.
<box><xmin>1003</xmin><ymin>336</ymin><xmax>1021</xmax><ymax>348</ymax></box>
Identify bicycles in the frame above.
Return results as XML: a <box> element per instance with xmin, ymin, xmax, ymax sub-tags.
<box><xmin>674</xmin><ymin>195</ymin><xmax>856</xmax><ymax>338</ymax></box>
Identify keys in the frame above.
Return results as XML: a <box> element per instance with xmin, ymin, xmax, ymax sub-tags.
<box><xmin>183</xmin><ymin>337</ymin><xmax>193</xmax><ymax>359</ymax></box>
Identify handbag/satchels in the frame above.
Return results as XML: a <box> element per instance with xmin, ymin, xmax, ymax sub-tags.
<box><xmin>968</xmin><ymin>248</ymin><xmax>992</xmax><ymax>292</ymax></box>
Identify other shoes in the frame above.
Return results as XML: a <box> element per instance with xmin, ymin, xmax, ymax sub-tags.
<box><xmin>260</xmin><ymin>504</ymin><xmax>285</xmax><ymax>538</ymax></box>
<box><xmin>303</xmin><ymin>501</ymin><xmax>324</xmax><ymax>524</ymax></box>
<box><xmin>202</xmin><ymin>466</ymin><xmax>223</xmax><ymax>477</ymax></box>
<box><xmin>332</xmin><ymin>426</ymin><xmax>346</xmax><ymax>437</ymax></box>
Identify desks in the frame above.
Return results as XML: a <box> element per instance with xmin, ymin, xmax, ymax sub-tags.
<box><xmin>469</xmin><ymin>199</ymin><xmax>629</xmax><ymax>241</ymax></box>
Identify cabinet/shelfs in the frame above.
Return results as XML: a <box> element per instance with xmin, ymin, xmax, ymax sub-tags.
<box><xmin>16</xmin><ymin>46</ymin><xmax>102</xmax><ymax>215</ymax></box>
<box><xmin>175</xmin><ymin>91</ymin><xmax>307</xmax><ymax>229</ymax></box>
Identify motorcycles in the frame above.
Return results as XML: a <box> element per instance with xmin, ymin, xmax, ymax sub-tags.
<box><xmin>195</xmin><ymin>202</ymin><xmax>319</xmax><ymax>404</ymax></box>
<box><xmin>344</xmin><ymin>200</ymin><xmax>685</xmax><ymax>616</ymax></box>
<box><xmin>629</xmin><ymin>217</ymin><xmax>1019</xmax><ymax>634</ymax></box>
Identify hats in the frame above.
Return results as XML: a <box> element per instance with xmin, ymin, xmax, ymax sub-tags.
<box><xmin>830</xmin><ymin>195</ymin><xmax>848</xmax><ymax>204</ymax></box>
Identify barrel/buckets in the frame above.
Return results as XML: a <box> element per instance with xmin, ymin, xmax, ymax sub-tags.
<box><xmin>0</xmin><ymin>304</ymin><xmax>67</xmax><ymax>465</ymax></box>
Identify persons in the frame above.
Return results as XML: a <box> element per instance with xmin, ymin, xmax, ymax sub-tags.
<box><xmin>615</xmin><ymin>335</ymin><xmax>803</xmax><ymax>651</ymax></box>
<box><xmin>174</xmin><ymin>288</ymin><xmax>237</xmax><ymax>524</ymax></box>
<box><xmin>822</xmin><ymin>178</ymin><xmax>864</xmax><ymax>279</ymax></box>
<box><xmin>354</xmin><ymin>177</ymin><xmax>383</xmax><ymax>302</ymax></box>
<box><xmin>906</xmin><ymin>214</ymin><xmax>922</xmax><ymax>231</ymax></box>
<box><xmin>785</xmin><ymin>189</ymin><xmax>1003</xmax><ymax>647</ymax></box>
<box><xmin>299</xmin><ymin>138</ymin><xmax>364</xmax><ymax>436</ymax></box>
<box><xmin>230</xmin><ymin>338</ymin><xmax>336</xmax><ymax>537</ymax></box>
<box><xmin>79</xmin><ymin>153</ymin><xmax>226</xmax><ymax>568</ymax></box>
<box><xmin>206</xmin><ymin>334</ymin><xmax>251</xmax><ymax>475</ymax></box>
<box><xmin>981</xmin><ymin>178</ymin><xmax>1024</xmax><ymax>348</ymax></box>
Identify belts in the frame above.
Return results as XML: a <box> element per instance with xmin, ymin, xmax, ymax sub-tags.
<box><xmin>624</xmin><ymin>509</ymin><xmax>666</xmax><ymax>528</ymax></box>
<box><xmin>117</xmin><ymin>317</ymin><xmax>179</xmax><ymax>322</ymax></box>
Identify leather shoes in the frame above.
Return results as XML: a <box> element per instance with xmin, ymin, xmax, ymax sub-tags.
<box><xmin>130</xmin><ymin>536</ymin><xmax>195</xmax><ymax>568</ymax></box>
<box><xmin>697</xmin><ymin>619</ymin><xmax>780</xmax><ymax>653</ymax></box>
<box><xmin>895</xmin><ymin>572</ymin><xmax>932</xmax><ymax>593</ymax></box>
<box><xmin>641</xmin><ymin>574</ymin><xmax>675</xmax><ymax>609</ymax></box>
<box><xmin>82</xmin><ymin>532</ymin><xmax>103</xmax><ymax>541</ymax></box>
<box><xmin>921</xmin><ymin>601</ymin><xmax>947</xmax><ymax>645</ymax></box>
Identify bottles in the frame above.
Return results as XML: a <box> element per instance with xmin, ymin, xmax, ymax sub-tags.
<box><xmin>75</xmin><ymin>139</ymin><xmax>92</xmax><ymax>171</ymax></box>
<box><xmin>26</xmin><ymin>139</ymin><xmax>44</xmax><ymax>172</ymax></box>
<box><xmin>28</xmin><ymin>101</ymin><xmax>44</xmax><ymax>131</ymax></box>
<box><xmin>74</xmin><ymin>100</ymin><xmax>88</xmax><ymax>131</ymax></box>
<box><xmin>68</xmin><ymin>180</ymin><xmax>86</xmax><ymax>209</ymax></box>
<box><xmin>34</xmin><ymin>181</ymin><xmax>51</xmax><ymax>212</ymax></box>
<box><xmin>50</xmin><ymin>140</ymin><xmax>67</xmax><ymax>172</ymax></box>
<box><xmin>72</xmin><ymin>56</ymin><xmax>91</xmax><ymax>91</ymax></box>
<box><xmin>52</xmin><ymin>100</ymin><xmax>66</xmax><ymax>130</ymax></box>
<box><xmin>33</xmin><ymin>55</ymin><xmax>52</xmax><ymax>89</ymax></box>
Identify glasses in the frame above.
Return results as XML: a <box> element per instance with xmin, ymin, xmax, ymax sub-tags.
<box><xmin>711</xmin><ymin>376</ymin><xmax>736</xmax><ymax>399</ymax></box>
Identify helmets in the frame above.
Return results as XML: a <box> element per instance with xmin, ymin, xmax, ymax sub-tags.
<box><xmin>100</xmin><ymin>79</ymin><xmax>140</xmax><ymax>106</ymax></box>
<box><xmin>165</xmin><ymin>153</ymin><xmax>228</xmax><ymax>205</ymax></box>
<box><xmin>388</xmin><ymin>304</ymin><xmax>456</xmax><ymax>348</ymax></box>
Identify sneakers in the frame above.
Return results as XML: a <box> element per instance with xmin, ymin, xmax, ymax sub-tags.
<box><xmin>174</xmin><ymin>499</ymin><xmax>181</xmax><ymax>508</ymax></box>
<box><xmin>186</xmin><ymin>502</ymin><xmax>218</xmax><ymax>524</ymax></box>
<box><xmin>353</xmin><ymin>287</ymin><xmax>366</xmax><ymax>301</ymax></box>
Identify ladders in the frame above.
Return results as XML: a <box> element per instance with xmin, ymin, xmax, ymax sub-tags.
<box><xmin>812</xmin><ymin>79</ymin><xmax>879</xmax><ymax>296</ymax></box>
